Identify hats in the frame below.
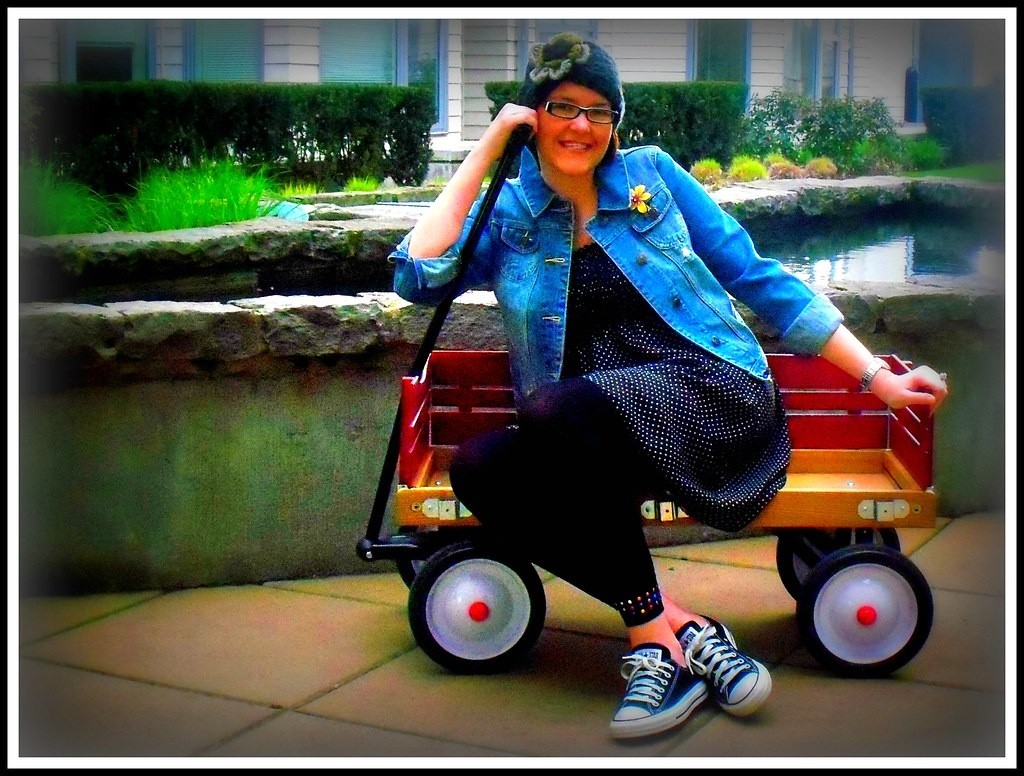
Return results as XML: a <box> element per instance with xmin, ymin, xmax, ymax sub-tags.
<box><xmin>517</xmin><ymin>32</ymin><xmax>625</xmax><ymax>129</ymax></box>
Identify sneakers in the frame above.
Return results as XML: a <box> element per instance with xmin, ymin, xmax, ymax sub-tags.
<box><xmin>607</xmin><ymin>642</ymin><xmax>709</xmax><ymax>738</ymax></box>
<box><xmin>673</xmin><ymin>613</ymin><xmax>772</xmax><ymax>717</ymax></box>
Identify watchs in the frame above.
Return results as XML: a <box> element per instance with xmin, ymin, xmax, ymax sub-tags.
<box><xmin>860</xmin><ymin>357</ymin><xmax>891</xmax><ymax>390</ymax></box>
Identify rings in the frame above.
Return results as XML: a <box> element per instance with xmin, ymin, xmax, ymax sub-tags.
<box><xmin>940</xmin><ymin>373</ymin><xmax>947</xmax><ymax>380</ymax></box>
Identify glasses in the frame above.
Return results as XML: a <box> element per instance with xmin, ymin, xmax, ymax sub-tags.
<box><xmin>539</xmin><ymin>100</ymin><xmax>620</xmax><ymax>124</ymax></box>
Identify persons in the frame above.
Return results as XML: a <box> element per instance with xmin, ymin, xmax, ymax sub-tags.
<box><xmin>388</xmin><ymin>31</ymin><xmax>948</xmax><ymax>737</ymax></box>
